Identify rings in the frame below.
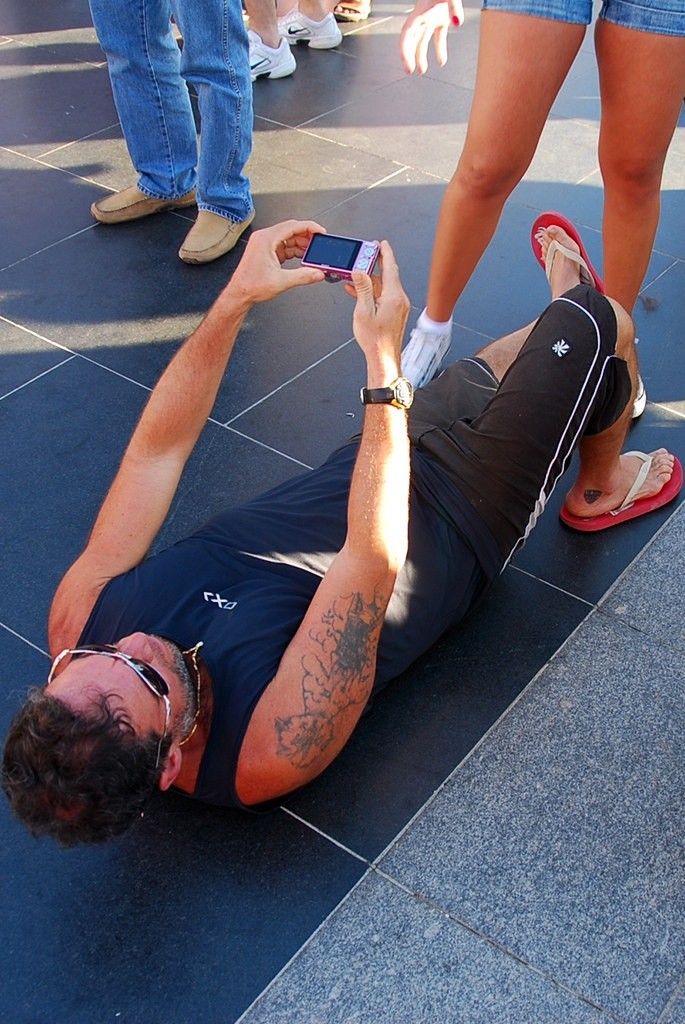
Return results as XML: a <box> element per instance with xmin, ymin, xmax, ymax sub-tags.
<box><xmin>282</xmin><ymin>240</ymin><xmax>288</xmax><ymax>248</ymax></box>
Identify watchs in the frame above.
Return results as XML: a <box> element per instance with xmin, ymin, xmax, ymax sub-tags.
<box><xmin>359</xmin><ymin>377</ymin><xmax>416</xmax><ymax>411</ymax></box>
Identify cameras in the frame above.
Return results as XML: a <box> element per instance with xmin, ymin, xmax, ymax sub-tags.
<box><xmin>300</xmin><ymin>233</ymin><xmax>381</xmax><ymax>283</ymax></box>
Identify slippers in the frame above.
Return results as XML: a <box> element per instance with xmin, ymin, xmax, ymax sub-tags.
<box><xmin>560</xmin><ymin>450</ymin><xmax>683</xmax><ymax>532</ymax></box>
<box><xmin>529</xmin><ymin>211</ymin><xmax>603</xmax><ymax>295</ymax></box>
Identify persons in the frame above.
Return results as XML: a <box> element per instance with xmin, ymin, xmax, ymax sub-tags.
<box><xmin>401</xmin><ymin>0</ymin><xmax>685</xmax><ymax>418</ymax></box>
<box><xmin>2</xmin><ymin>210</ymin><xmax>684</xmax><ymax>849</ymax></box>
<box><xmin>90</xmin><ymin>0</ymin><xmax>371</xmax><ymax>263</ymax></box>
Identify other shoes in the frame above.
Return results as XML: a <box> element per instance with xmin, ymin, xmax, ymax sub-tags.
<box><xmin>179</xmin><ymin>208</ymin><xmax>256</xmax><ymax>264</ymax></box>
<box><xmin>89</xmin><ymin>184</ymin><xmax>197</xmax><ymax>224</ymax></box>
<box><xmin>632</xmin><ymin>374</ymin><xmax>646</xmax><ymax>418</ymax></box>
<box><xmin>400</xmin><ymin>306</ymin><xmax>452</xmax><ymax>393</ymax></box>
<box><xmin>333</xmin><ymin>0</ymin><xmax>371</xmax><ymax>22</ymax></box>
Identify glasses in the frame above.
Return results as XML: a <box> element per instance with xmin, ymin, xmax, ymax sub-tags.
<box><xmin>48</xmin><ymin>644</ymin><xmax>171</xmax><ymax>738</ymax></box>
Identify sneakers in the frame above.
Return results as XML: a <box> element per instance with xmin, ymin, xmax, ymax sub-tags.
<box><xmin>247</xmin><ymin>30</ymin><xmax>296</xmax><ymax>82</ymax></box>
<box><xmin>277</xmin><ymin>2</ymin><xmax>342</xmax><ymax>49</ymax></box>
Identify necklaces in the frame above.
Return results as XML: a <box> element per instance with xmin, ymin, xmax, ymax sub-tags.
<box><xmin>179</xmin><ymin>641</ymin><xmax>204</xmax><ymax>748</ymax></box>
